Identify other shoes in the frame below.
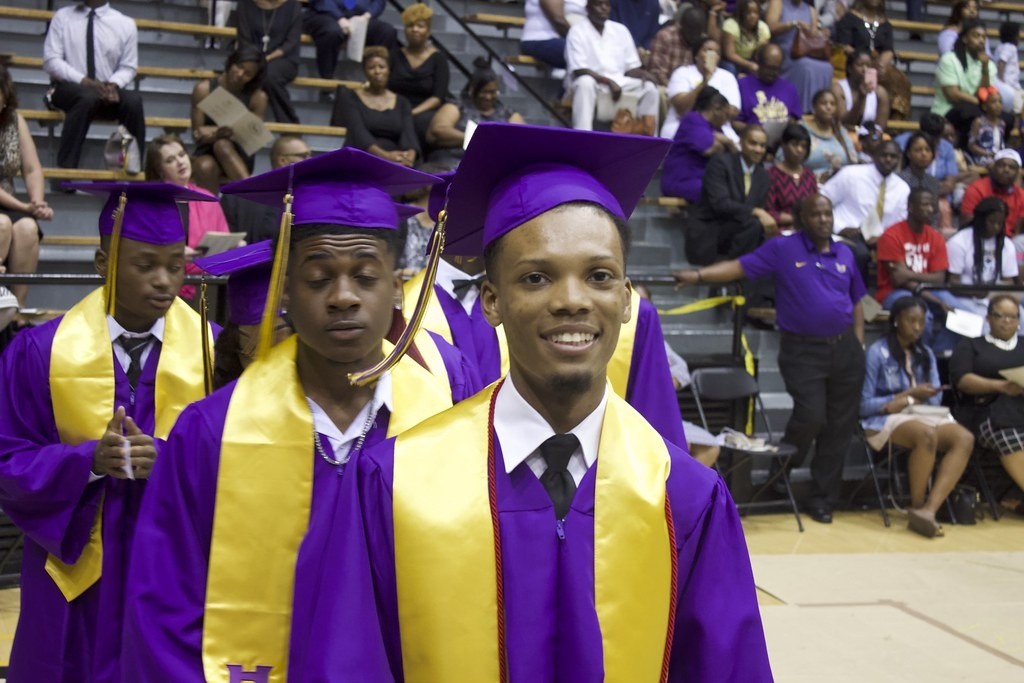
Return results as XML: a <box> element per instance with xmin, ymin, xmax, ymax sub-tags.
<box><xmin>809</xmin><ymin>506</ymin><xmax>832</xmax><ymax>523</ymax></box>
<box><xmin>709</xmin><ymin>286</ymin><xmax>726</xmax><ymax>301</ymax></box>
<box><xmin>907</xmin><ymin>508</ymin><xmax>936</xmax><ymax>538</ymax></box>
<box><xmin>770</xmin><ymin>456</ymin><xmax>792</xmax><ymax>500</ymax></box>
<box><xmin>318</xmin><ymin>92</ymin><xmax>336</xmax><ymax>103</ymax></box>
<box><xmin>935</xmin><ymin>521</ymin><xmax>945</xmax><ymax>536</ymax></box>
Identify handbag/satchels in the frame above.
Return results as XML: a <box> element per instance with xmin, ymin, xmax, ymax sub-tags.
<box><xmin>936</xmin><ymin>483</ymin><xmax>984</xmax><ymax>524</ymax></box>
<box><xmin>610</xmin><ymin>109</ymin><xmax>657</xmax><ymax>136</ymax></box>
<box><xmin>790</xmin><ymin>26</ymin><xmax>832</xmax><ymax>61</ymax></box>
<box><xmin>103</xmin><ymin>125</ymin><xmax>141</xmax><ymax>176</ymax></box>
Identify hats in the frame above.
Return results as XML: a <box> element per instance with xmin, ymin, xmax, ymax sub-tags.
<box><xmin>220</xmin><ymin>148</ymin><xmax>444</xmax><ymax>363</ymax></box>
<box><xmin>427</xmin><ymin>171</ymin><xmax>454</xmax><ymax>223</ymax></box>
<box><xmin>346</xmin><ymin>121</ymin><xmax>675</xmax><ymax>389</ymax></box>
<box><xmin>994</xmin><ymin>149</ymin><xmax>1022</xmax><ymax>168</ymax></box>
<box><xmin>393</xmin><ymin>200</ymin><xmax>425</xmax><ymax>221</ymax></box>
<box><xmin>192</xmin><ymin>238</ymin><xmax>287</xmax><ymax>324</ymax></box>
<box><xmin>59</xmin><ymin>182</ymin><xmax>220</xmax><ymax>319</ymax></box>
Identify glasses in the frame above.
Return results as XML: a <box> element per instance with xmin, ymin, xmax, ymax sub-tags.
<box><xmin>989</xmin><ymin>312</ymin><xmax>1019</xmax><ymax>321</ymax></box>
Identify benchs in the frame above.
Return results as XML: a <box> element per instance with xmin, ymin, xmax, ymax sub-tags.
<box><xmin>0</xmin><ymin>0</ymin><xmax>1024</xmax><ymax>482</ymax></box>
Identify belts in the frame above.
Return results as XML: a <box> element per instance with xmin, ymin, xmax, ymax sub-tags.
<box><xmin>781</xmin><ymin>329</ymin><xmax>855</xmax><ymax>344</ymax></box>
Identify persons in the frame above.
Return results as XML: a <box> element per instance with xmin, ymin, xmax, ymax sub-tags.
<box><xmin>0</xmin><ymin>0</ymin><xmax>1024</xmax><ymax>540</ymax></box>
<box><xmin>120</xmin><ymin>148</ymin><xmax>463</xmax><ymax>683</ymax></box>
<box><xmin>0</xmin><ymin>181</ymin><xmax>224</xmax><ymax>683</ymax></box>
<box><xmin>286</xmin><ymin>121</ymin><xmax>774</xmax><ymax>683</ymax></box>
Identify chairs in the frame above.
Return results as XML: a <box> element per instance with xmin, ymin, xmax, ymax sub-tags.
<box><xmin>684</xmin><ymin>369</ymin><xmax>808</xmax><ymax>533</ymax></box>
<box><xmin>850</xmin><ymin>356</ymin><xmax>1015</xmax><ymax>527</ymax></box>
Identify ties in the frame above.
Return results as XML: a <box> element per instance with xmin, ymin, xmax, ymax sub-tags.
<box><xmin>118</xmin><ymin>334</ymin><xmax>154</xmax><ymax>393</ymax></box>
<box><xmin>877</xmin><ymin>179</ymin><xmax>885</xmax><ymax>222</ymax></box>
<box><xmin>539</xmin><ymin>433</ymin><xmax>580</xmax><ymax>523</ymax></box>
<box><xmin>744</xmin><ymin>170</ymin><xmax>752</xmax><ymax>195</ymax></box>
<box><xmin>87</xmin><ymin>10</ymin><xmax>96</xmax><ymax>80</ymax></box>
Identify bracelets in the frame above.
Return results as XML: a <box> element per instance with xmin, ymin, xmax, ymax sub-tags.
<box><xmin>697</xmin><ymin>270</ymin><xmax>702</xmax><ymax>281</ymax></box>
<box><xmin>907</xmin><ymin>395</ymin><xmax>915</xmax><ymax>406</ymax></box>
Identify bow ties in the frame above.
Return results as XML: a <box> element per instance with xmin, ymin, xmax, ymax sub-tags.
<box><xmin>452</xmin><ymin>275</ymin><xmax>487</xmax><ymax>300</ymax></box>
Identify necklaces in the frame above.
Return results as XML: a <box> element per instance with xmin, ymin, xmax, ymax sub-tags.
<box><xmin>306</xmin><ymin>398</ymin><xmax>373</xmax><ymax>466</ymax></box>
